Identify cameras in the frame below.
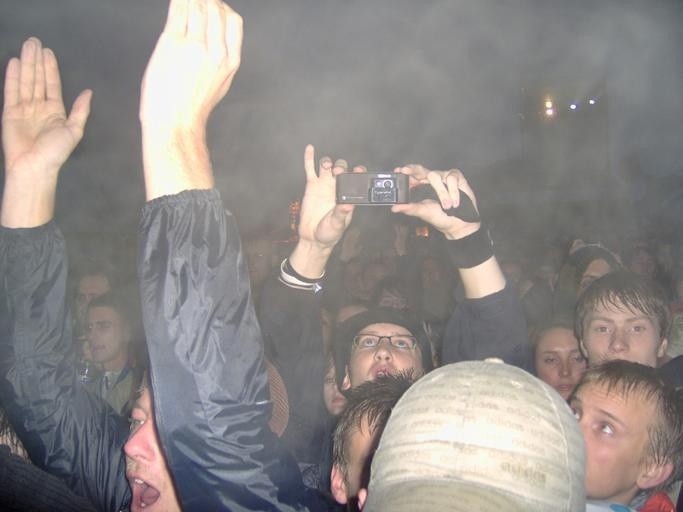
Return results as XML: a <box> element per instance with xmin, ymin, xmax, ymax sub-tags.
<box><xmin>336</xmin><ymin>172</ymin><xmax>410</xmax><ymax>205</ymax></box>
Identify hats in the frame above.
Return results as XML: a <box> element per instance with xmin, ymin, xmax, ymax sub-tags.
<box><xmin>358</xmin><ymin>355</ymin><xmax>589</xmax><ymax>512</ymax></box>
<box><xmin>330</xmin><ymin>306</ymin><xmax>434</xmax><ymax>404</ymax></box>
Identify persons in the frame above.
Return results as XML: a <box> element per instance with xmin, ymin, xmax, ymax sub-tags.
<box><xmin>1</xmin><ymin>140</ymin><xmax>683</xmax><ymax>512</ymax></box>
<box><xmin>0</xmin><ymin>0</ymin><xmax>290</xmax><ymax>512</ymax></box>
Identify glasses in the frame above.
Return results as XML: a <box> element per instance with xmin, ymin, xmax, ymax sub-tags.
<box><xmin>350</xmin><ymin>332</ymin><xmax>422</xmax><ymax>350</ymax></box>
